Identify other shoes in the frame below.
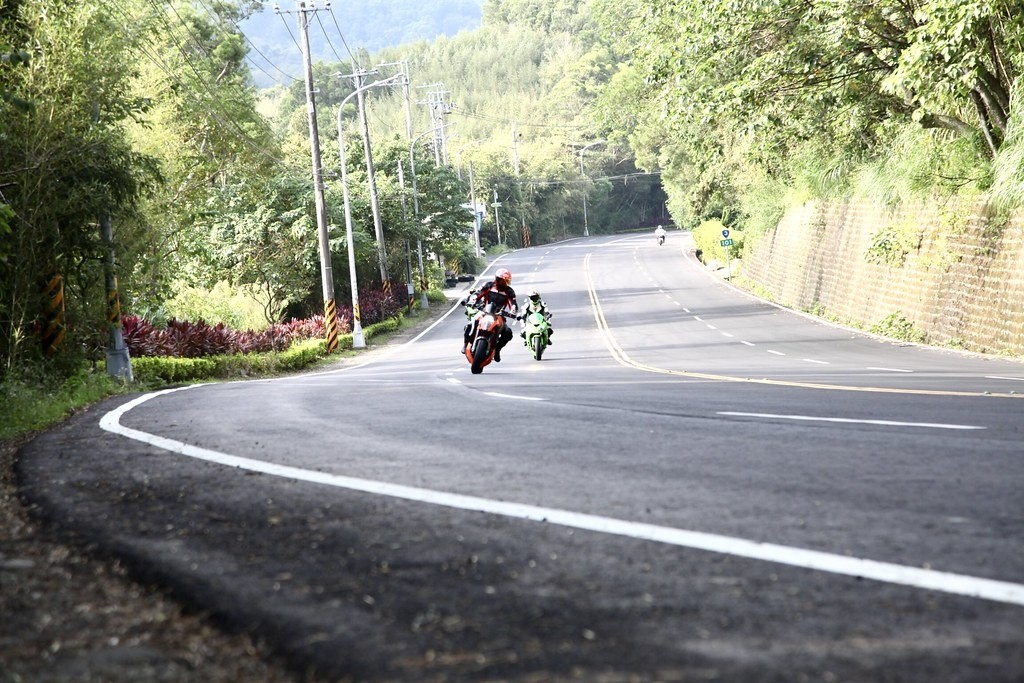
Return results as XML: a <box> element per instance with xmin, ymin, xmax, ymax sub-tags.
<box><xmin>548</xmin><ymin>339</ymin><xmax>552</xmax><ymax>345</ymax></box>
<box><xmin>525</xmin><ymin>341</ymin><xmax>527</xmax><ymax>347</ymax></box>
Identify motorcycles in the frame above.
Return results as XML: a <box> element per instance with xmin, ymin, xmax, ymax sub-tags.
<box><xmin>656</xmin><ymin>228</ymin><xmax>667</xmax><ymax>246</ymax></box>
<box><xmin>461</xmin><ymin>300</ymin><xmax>519</xmax><ymax>375</ymax></box>
<box><xmin>515</xmin><ymin>313</ymin><xmax>554</xmax><ymax>362</ymax></box>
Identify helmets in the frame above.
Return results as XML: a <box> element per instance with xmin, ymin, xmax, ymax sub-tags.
<box><xmin>495</xmin><ymin>268</ymin><xmax>512</xmax><ymax>285</ymax></box>
<box><xmin>529</xmin><ymin>289</ymin><xmax>541</xmax><ymax>304</ymax></box>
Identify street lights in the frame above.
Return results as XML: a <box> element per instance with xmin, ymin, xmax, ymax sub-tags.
<box><xmin>457</xmin><ymin>135</ymin><xmax>492</xmax><ymax>195</ymax></box>
<box><xmin>406</xmin><ymin>119</ymin><xmax>456</xmax><ymax>309</ymax></box>
<box><xmin>336</xmin><ymin>70</ymin><xmax>406</xmax><ymax>350</ymax></box>
<box><xmin>579</xmin><ymin>138</ymin><xmax>608</xmax><ymax>233</ymax></box>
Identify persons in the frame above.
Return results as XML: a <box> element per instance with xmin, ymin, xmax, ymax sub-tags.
<box><xmin>655</xmin><ymin>225</ymin><xmax>666</xmax><ymax>243</ymax></box>
<box><xmin>520</xmin><ymin>292</ymin><xmax>554</xmax><ymax>347</ymax></box>
<box><xmin>461</xmin><ymin>268</ymin><xmax>519</xmax><ymax>362</ymax></box>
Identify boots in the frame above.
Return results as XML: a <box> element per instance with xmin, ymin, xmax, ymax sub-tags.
<box><xmin>461</xmin><ymin>326</ymin><xmax>470</xmax><ymax>354</ymax></box>
<box><xmin>494</xmin><ymin>340</ymin><xmax>507</xmax><ymax>362</ymax></box>
